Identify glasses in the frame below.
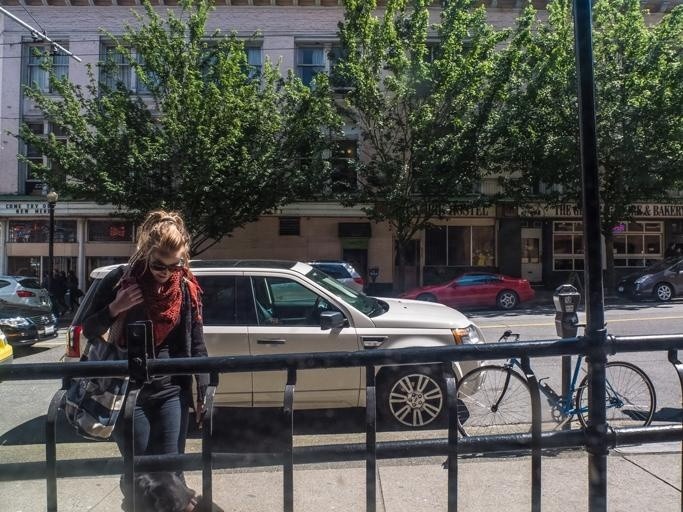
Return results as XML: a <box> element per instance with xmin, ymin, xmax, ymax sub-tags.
<box><xmin>149</xmin><ymin>260</ymin><xmax>183</xmax><ymax>274</ymax></box>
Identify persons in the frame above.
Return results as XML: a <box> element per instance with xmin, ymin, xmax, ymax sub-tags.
<box><xmin>40</xmin><ymin>266</ymin><xmax>80</xmax><ymax>317</ymax></box>
<box><xmin>81</xmin><ymin>212</ymin><xmax>212</xmax><ymax>510</ymax></box>
<box><xmin>15</xmin><ymin>226</ymin><xmax>75</xmax><ymax>242</ymax></box>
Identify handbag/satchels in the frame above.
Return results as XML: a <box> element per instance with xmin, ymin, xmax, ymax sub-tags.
<box><xmin>60</xmin><ymin>264</ymin><xmax>135</xmax><ymax>443</ymax></box>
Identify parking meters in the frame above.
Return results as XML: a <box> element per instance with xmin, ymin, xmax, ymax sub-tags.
<box><xmin>550</xmin><ymin>283</ymin><xmax>583</xmax><ymax>429</ymax></box>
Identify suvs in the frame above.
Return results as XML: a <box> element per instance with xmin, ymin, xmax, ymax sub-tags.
<box><xmin>215</xmin><ymin>258</ymin><xmax>365</xmax><ymax>308</ymax></box>
<box><xmin>61</xmin><ymin>262</ymin><xmax>489</xmax><ymax>433</ymax></box>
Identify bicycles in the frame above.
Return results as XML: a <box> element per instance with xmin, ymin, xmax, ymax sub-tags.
<box><xmin>450</xmin><ymin>322</ymin><xmax>657</xmax><ymax>445</ymax></box>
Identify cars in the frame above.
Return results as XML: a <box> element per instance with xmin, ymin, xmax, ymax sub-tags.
<box><xmin>619</xmin><ymin>255</ymin><xmax>682</xmax><ymax>304</ymax></box>
<box><xmin>396</xmin><ymin>271</ymin><xmax>533</xmax><ymax>313</ymax></box>
<box><xmin>0</xmin><ymin>274</ymin><xmax>58</xmax><ymax>367</ymax></box>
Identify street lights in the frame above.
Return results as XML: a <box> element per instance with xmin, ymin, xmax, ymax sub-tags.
<box><xmin>46</xmin><ymin>191</ymin><xmax>59</xmax><ymax>314</ymax></box>
